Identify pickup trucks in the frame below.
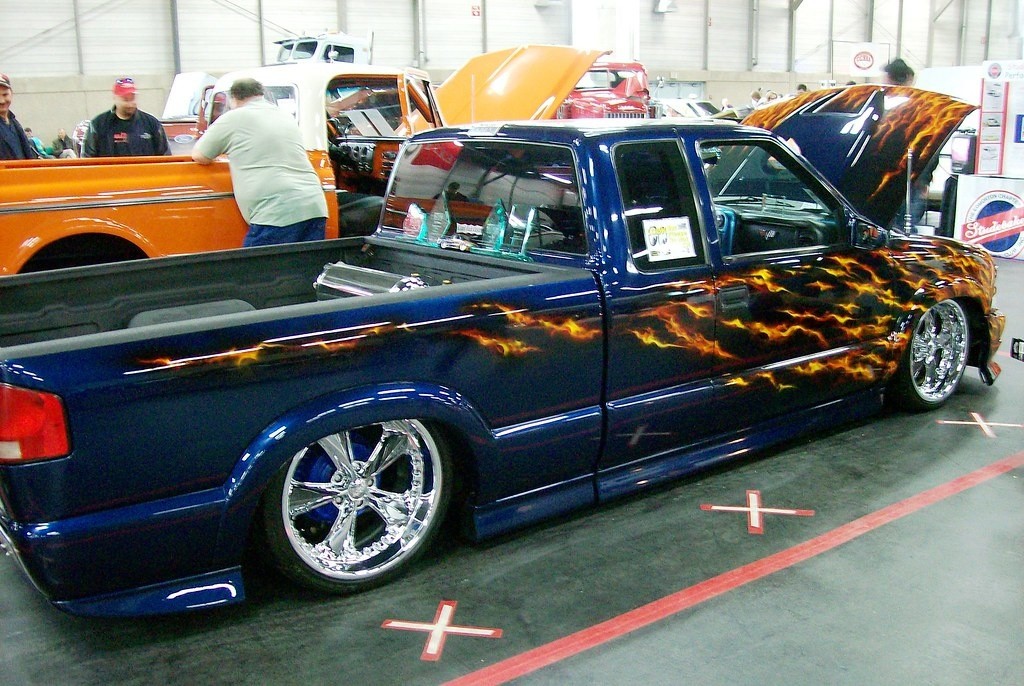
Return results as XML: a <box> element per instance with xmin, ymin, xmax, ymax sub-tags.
<box><xmin>0</xmin><ymin>83</ymin><xmax>1007</xmax><ymax>619</ymax></box>
<box><xmin>159</xmin><ymin>119</ymin><xmax>204</xmax><ymax>146</ymax></box>
<box><xmin>0</xmin><ymin>42</ymin><xmax>611</xmax><ymax>278</ymax></box>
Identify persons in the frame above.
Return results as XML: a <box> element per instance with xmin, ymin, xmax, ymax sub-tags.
<box><xmin>81</xmin><ymin>77</ymin><xmax>172</xmax><ymax>158</ymax></box>
<box><xmin>0</xmin><ymin>71</ymin><xmax>34</xmax><ymax>159</ymax></box>
<box><xmin>750</xmin><ymin>87</ymin><xmax>782</xmax><ymax>109</ymax></box>
<box><xmin>797</xmin><ymin>84</ymin><xmax>807</xmax><ymax>94</ymax></box>
<box><xmin>846</xmin><ymin>81</ymin><xmax>856</xmax><ymax>85</ymax></box>
<box><xmin>721</xmin><ymin>98</ymin><xmax>734</xmax><ymax>111</ymax></box>
<box><xmin>190</xmin><ymin>77</ymin><xmax>329</xmax><ymax>248</ymax></box>
<box><xmin>883</xmin><ymin>58</ymin><xmax>942</xmax><ymax>236</ymax></box>
<box><xmin>52</xmin><ymin>128</ymin><xmax>77</xmax><ymax>159</ymax></box>
<box><xmin>24</xmin><ymin>127</ymin><xmax>54</xmax><ymax>159</ymax></box>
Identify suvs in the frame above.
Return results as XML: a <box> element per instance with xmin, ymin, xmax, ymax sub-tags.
<box><xmin>555</xmin><ymin>63</ymin><xmax>654</xmax><ymax>119</ymax></box>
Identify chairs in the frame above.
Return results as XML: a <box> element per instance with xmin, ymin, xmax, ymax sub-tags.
<box><xmin>338</xmin><ymin>172</ymin><xmax>385</xmax><ymax>236</ymax></box>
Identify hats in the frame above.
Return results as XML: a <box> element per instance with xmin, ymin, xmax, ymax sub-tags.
<box><xmin>0</xmin><ymin>73</ymin><xmax>11</xmax><ymax>89</ymax></box>
<box><xmin>114</xmin><ymin>78</ymin><xmax>136</xmax><ymax>95</ymax></box>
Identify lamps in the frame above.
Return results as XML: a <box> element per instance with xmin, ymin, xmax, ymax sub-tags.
<box><xmin>654</xmin><ymin>0</ymin><xmax>675</xmax><ymax>14</ymax></box>
